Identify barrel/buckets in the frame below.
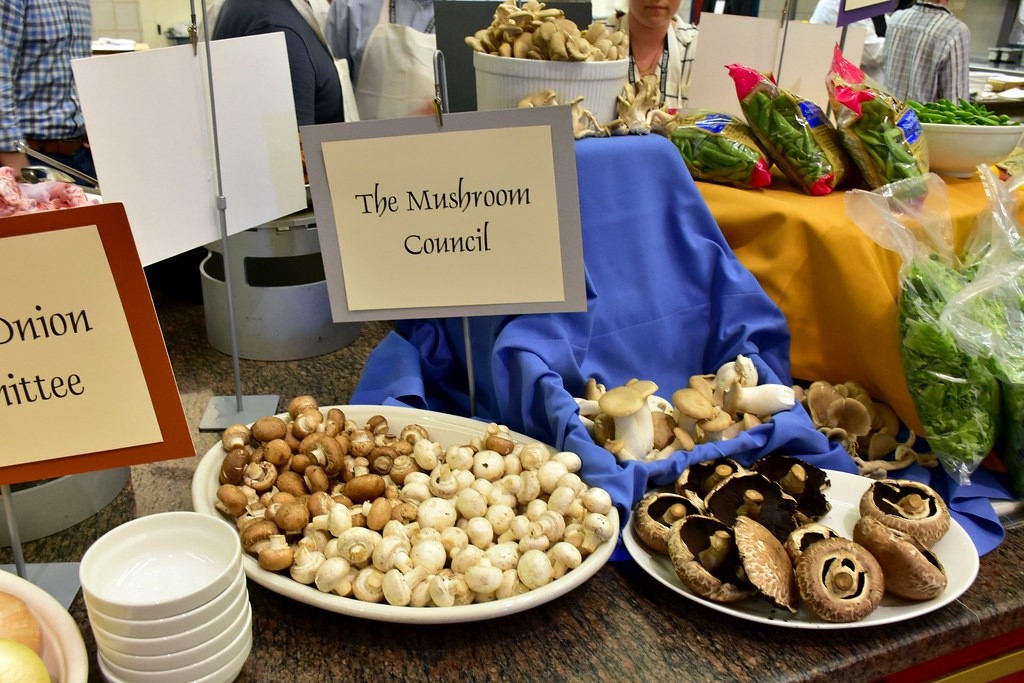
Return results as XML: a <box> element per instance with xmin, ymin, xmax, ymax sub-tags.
<box><xmin>473</xmin><ymin>50</ymin><xmax>629</xmax><ymax>128</ymax></box>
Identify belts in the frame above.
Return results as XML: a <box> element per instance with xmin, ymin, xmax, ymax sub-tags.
<box><xmin>25</xmin><ymin>134</ymin><xmax>86</xmax><ymax>154</ymax></box>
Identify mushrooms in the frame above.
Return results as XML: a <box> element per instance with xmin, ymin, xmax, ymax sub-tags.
<box><xmin>213</xmin><ymin>395</ymin><xmax>614</xmax><ymax>607</ymax></box>
<box><xmin>572</xmin><ymin>352</ymin><xmax>951</xmax><ymax>622</ymax></box>
<box><xmin>518</xmin><ymin>73</ymin><xmax>682</xmax><ymax>139</ymax></box>
<box><xmin>464</xmin><ymin>0</ymin><xmax>629</xmax><ymax>65</ymax></box>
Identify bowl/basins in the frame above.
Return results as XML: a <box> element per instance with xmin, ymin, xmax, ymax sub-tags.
<box><xmin>1</xmin><ymin>568</ymin><xmax>90</xmax><ymax>683</ymax></box>
<box><xmin>910</xmin><ymin>121</ymin><xmax>1024</xmax><ymax>179</ymax></box>
<box><xmin>74</xmin><ymin>510</ymin><xmax>254</xmax><ymax>683</ymax></box>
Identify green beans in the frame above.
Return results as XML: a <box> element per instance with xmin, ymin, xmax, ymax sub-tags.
<box><xmin>667</xmin><ymin>129</ymin><xmax>760</xmax><ymax>186</ymax></box>
<box><xmin>743</xmin><ymin>88</ymin><xmax>832</xmax><ymax>189</ymax></box>
<box><xmin>904</xmin><ymin>96</ymin><xmax>1020</xmax><ymax>126</ymax></box>
<box><xmin>848</xmin><ymin>96</ymin><xmax>925</xmax><ymax>202</ymax></box>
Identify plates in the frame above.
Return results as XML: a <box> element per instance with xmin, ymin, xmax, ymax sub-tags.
<box><xmin>622</xmin><ymin>464</ymin><xmax>980</xmax><ymax>630</ymax></box>
<box><xmin>192</xmin><ymin>404</ymin><xmax>619</xmax><ymax>624</ymax></box>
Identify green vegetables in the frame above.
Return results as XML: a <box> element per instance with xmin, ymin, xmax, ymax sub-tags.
<box><xmin>899</xmin><ymin>240</ymin><xmax>1024</xmax><ymax>506</ymax></box>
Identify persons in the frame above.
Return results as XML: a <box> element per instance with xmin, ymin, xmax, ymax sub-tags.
<box><xmin>879</xmin><ymin>0</ymin><xmax>973</xmax><ymax>115</ymax></box>
<box><xmin>591</xmin><ymin>0</ymin><xmax>705</xmax><ymax>139</ymax></box>
<box><xmin>809</xmin><ymin>0</ymin><xmax>886</xmax><ymax>94</ymax></box>
<box><xmin>0</xmin><ymin>0</ymin><xmax>96</xmax><ymax>186</ymax></box>
<box><xmin>323</xmin><ymin>0</ymin><xmax>446</xmax><ymax>128</ymax></box>
<box><xmin>197</xmin><ymin>0</ymin><xmax>347</xmax><ymax>185</ymax></box>
